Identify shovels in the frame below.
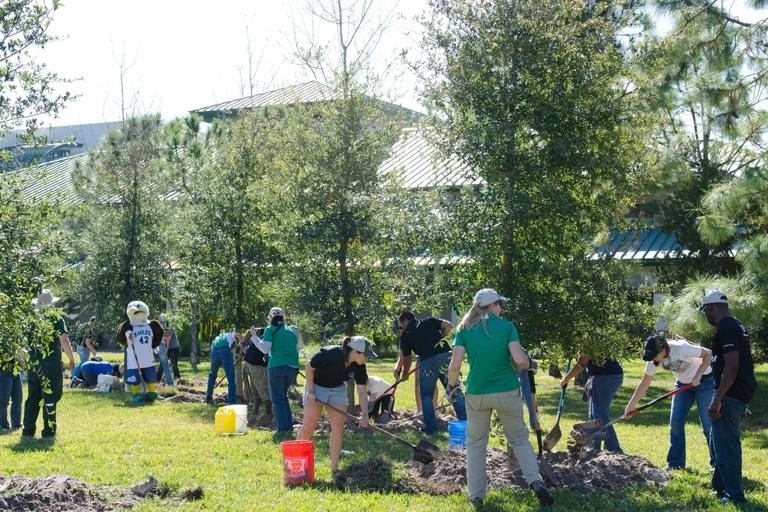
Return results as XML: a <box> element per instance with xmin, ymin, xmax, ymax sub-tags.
<box><xmin>528</xmin><ymin>371</ymin><xmax>559</xmax><ymax>486</ymax></box>
<box><xmin>544</xmin><ymin>361</ymin><xmax>570</xmax><ymax>451</ymax></box>
<box><xmin>378</xmin><ymin>363</ymin><xmax>403</xmax><ymax>424</ymax></box>
<box><xmin>573</xmin><ymin>383</ymin><xmax>692</xmax><ymax>440</ymax></box>
<box><xmin>315</xmin><ymin>399</ymin><xmax>440</xmax><ymax>465</ymax></box>
<box><xmin>355</xmin><ymin>365</ymin><xmax>420</xmax><ymax>415</ymax></box>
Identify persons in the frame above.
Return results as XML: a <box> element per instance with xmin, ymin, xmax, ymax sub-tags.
<box><xmin>622</xmin><ymin>334</ymin><xmax>718</xmax><ymax>471</ymax></box>
<box><xmin>560</xmin><ymin>338</ymin><xmax>623</xmax><ymax>456</ymax></box>
<box><xmin>294</xmin><ymin>335</ymin><xmax>377</xmax><ymax>478</ymax></box>
<box><xmin>392</xmin><ymin>318</ymin><xmax>438</xmax><ymax>416</ymax></box>
<box><xmin>22</xmin><ymin>289</ymin><xmax>75</xmax><ymax>439</ymax></box>
<box><xmin>694</xmin><ymin>290</ymin><xmax>757</xmax><ymax>505</ymax></box>
<box><xmin>235</xmin><ymin>325</ymin><xmax>300</xmax><ymax>420</ymax></box>
<box><xmin>203</xmin><ymin>332</ymin><xmax>244</xmax><ymax>406</ymax></box>
<box><xmin>155</xmin><ymin>314</ymin><xmax>181</xmax><ymax>384</ymax></box>
<box><xmin>444</xmin><ymin>288</ymin><xmax>554</xmax><ymax>510</ymax></box>
<box><xmin>365</xmin><ymin>373</ymin><xmax>394</xmax><ymax>418</ymax></box>
<box><xmin>249</xmin><ymin>306</ymin><xmax>299</xmax><ymax>434</ymax></box>
<box><xmin>76</xmin><ymin>315</ymin><xmax>97</xmax><ymax>363</ymax></box>
<box><xmin>397</xmin><ymin>310</ymin><xmax>466</xmax><ymax>433</ymax></box>
<box><xmin>0</xmin><ymin>345</ymin><xmax>28</xmax><ymax>434</ymax></box>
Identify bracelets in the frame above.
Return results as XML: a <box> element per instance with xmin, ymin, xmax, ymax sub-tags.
<box><xmin>307</xmin><ymin>389</ymin><xmax>316</xmax><ymax>395</ymax></box>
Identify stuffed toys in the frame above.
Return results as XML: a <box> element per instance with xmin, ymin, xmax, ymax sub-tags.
<box><xmin>115</xmin><ymin>300</ymin><xmax>163</xmax><ymax>404</ymax></box>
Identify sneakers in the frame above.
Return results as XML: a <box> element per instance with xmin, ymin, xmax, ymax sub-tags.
<box><xmin>70</xmin><ymin>376</ymin><xmax>78</xmax><ymax>388</ymax></box>
<box><xmin>533</xmin><ymin>482</ymin><xmax>553</xmax><ymax>505</ymax></box>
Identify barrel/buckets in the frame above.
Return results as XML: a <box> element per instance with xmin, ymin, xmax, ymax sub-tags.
<box><xmin>215</xmin><ymin>406</ymin><xmax>237</xmax><ymax>437</ymax></box>
<box><xmin>97</xmin><ymin>374</ymin><xmax>114</xmax><ymax>392</ymax></box>
<box><xmin>223</xmin><ymin>403</ymin><xmax>248</xmax><ymax>433</ymax></box>
<box><xmin>447</xmin><ymin>419</ymin><xmax>468</xmax><ymax>449</ymax></box>
<box><xmin>281</xmin><ymin>439</ymin><xmax>315</xmax><ymax>487</ymax></box>
<box><xmin>125</xmin><ymin>372</ymin><xmax>145</xmax><ymax>394</ymax></box>
<box><xmin>18</xmin><ymin>370</ymin><xmax>29</xmax><ymax>380</ymax></box>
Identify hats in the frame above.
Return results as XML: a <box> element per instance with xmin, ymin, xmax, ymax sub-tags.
<box><xmin>268</xmin><ymin>307</ymin><xmax>283</xmax><ymax>319</ymax></box>
<box><xmin>703</xmin><ymin>290</ymin><xmax>728</xmax><ymax>305</ymax></box>
<box><xmin>347</xmin><ymin>336</ymin><xmax>378</xmax><ymax>359</ymax></box>
<box><xmin>474</xmin><ymin>289</ymin><xmax>508</xmax><ymax>306</ymax></box>
<box><xmin>643</xmin><ymin>338</ymin><xmax>662</xmax><ymax>361</ymax></box>
<box><xmin>32</xmin><ymin>289</ymin><xmax>59</xmax><ymax>306</ymax></box>
